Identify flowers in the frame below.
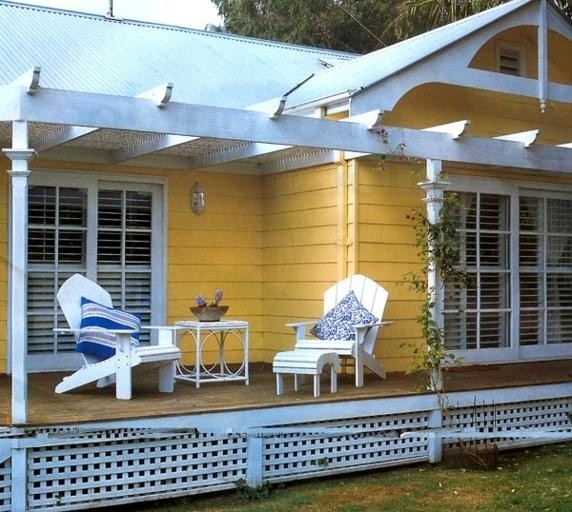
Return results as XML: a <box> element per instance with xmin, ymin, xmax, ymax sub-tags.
<box><xmin>195</xmin><ymin>289</ymin><xmax>224</xmax><ymax>307</ymax></box>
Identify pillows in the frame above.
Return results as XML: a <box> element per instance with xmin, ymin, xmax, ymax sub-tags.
<box><xmin>76</xmin><ymin>296</ymin><xmax>141</xmax><ymax>360</ymax></box>
<box><xmin>309</xmin><ymin>289</ymin><xmax>380</xmax><ymax>341</ymax></box>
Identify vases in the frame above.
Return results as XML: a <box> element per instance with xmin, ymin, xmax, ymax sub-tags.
<box><xmin>189</xmin><ymin>306</ymin><xmax>229</xmax><ymax>322</ymax></box>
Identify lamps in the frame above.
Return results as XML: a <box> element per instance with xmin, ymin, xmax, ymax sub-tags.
<box><xmin>190</xmin><ymin>182</ymin><xmax>206</xmax><ymax>216</ymax></box>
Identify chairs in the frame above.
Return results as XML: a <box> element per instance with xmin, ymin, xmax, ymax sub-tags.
<box><xmin>271</xmin><ymin>272</ymin><xmax>395</xmax><ymax>398</ymax></box>
<box><xmin>51</xmin><ymin>272</ymin><xmax>185</xmax><ymax>401</ymax></box>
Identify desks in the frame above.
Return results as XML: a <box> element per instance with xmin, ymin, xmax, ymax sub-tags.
<box><xmin>172</xmin><ymin>318</ymin><xmax>250</xmax><ymax>389</ymax></box>
